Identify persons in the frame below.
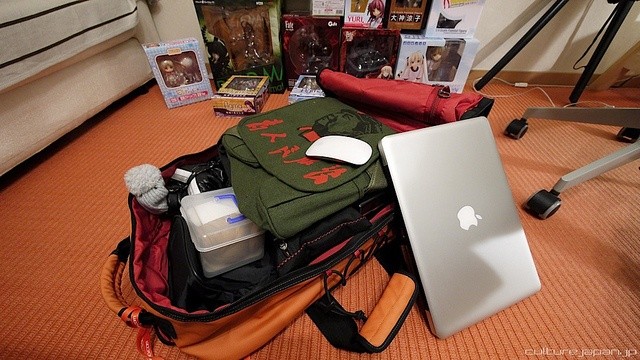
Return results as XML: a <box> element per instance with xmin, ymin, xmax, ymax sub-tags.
<box><xmin>140</xmin><ymin>37</ymin><xmax>215</xmax><ymax>111</ymax></box>
<box><xmin>392</xmin><ymin>32</ymin><xmax>480</xmax><ymax>94</ymax></box>
<box><xmin>288</xmin><ymin>74</ymin><xmax>327</xmax><ymax>103</ymax></box>
<box><xmin>211</xmin><ymin>74</ymin><xmax>270</xmax><ymax>118</ymax></box>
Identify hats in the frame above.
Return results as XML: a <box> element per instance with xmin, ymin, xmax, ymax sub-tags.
<box><xmin>123</xmin><ymin>163</ymin><xmax>169</xmax><ymax>213</ymax></box>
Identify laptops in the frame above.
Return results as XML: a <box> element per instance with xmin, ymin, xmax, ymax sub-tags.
<box><xmin>376</xmin><ymin>114</ymin><xmax>545</xmax><ymax>340</ymax></box>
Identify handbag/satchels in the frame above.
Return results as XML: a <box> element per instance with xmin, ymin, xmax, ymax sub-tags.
<box><xmin>101</xmin><ymin>67</ymin><xmax>494</xmax><ymax>360</ymax></box>
<box><xmin>166</xmin><ymin>214</ymin><xmax>278</xmax><ymax>311</ymax></box>
<box><xmin>218</xmin><ymin>95</ymin><xmax>402</xmax><ymax>239</ymax></box>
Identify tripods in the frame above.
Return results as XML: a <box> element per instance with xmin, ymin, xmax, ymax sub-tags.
<box><xmin>474</xmin><ymin>0</ymin><xmax>640</xmax><ymax>104</ymax></box>
